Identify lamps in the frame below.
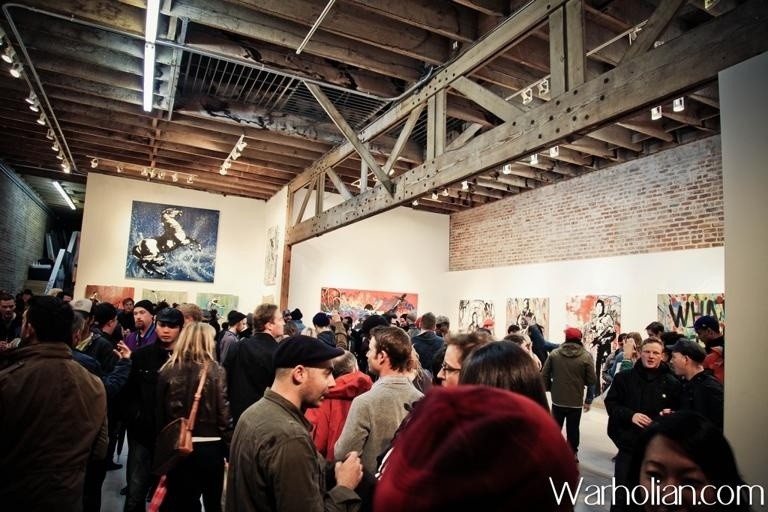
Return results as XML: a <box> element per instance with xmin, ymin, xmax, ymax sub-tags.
<box><xmin>84</xmin><ymin>154</ymin><xmax>198</xmax><ymax>187</ymax></box>
<box><xmin>409</xmin><ymin>179</ymin><xmax>470</xmax><ymax>207</ymax></box>
<box><xmin>628</xmin><ymin>26</ymin><xmax>665</xmax><ymax>48</ymax></box>
<box><xmin>519</xmin><ymin>77</ymin><xmax>550</xmax><ymax>103</ymax></box>
<box><xmin>0</xmin><ymin>1</ymin><xmax>77</xmax><ymax>178</ymax></box>
<box><xmin>502</xmin><ymin>146</ymin><xmax>562</xmax><ymax>176</ymax></box>
<box><xmin>650</xmin><ymin>96</ymin><xmax>685</xmax><ymax>121</ymax></box>
<box><xmin>216</xmin><ymin>133</ymin><xmax>248</xmax><ymax>177</ymax></box>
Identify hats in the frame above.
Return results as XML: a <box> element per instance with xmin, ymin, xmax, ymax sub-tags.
<box><xmin>665</xmin><ymin>314</ymin><xmax>721</xmax><ymax>363</ymax></box>
<box><xmin>270</xmin><ymin>334</ymin><xmax>346</xmax><ymax>368</ymax></box>
<box><xmin>156</xmin><ymin>307</ymin><xmax>184</xmax><ymax>323</ymax></box>
<box><xmin>563</xmin><ymin>327</ymin><xmax>582</xmax><ymax>341</ymax></box>
<box><xmin>134</xmin><ymin>300</ymin><xmax>155</xmax><ymax>315</ymax></box>
<box><xmin>68</xmin><ymin>297</ymin><xmax>95</xmax><ymax>315</ymax></box>
<box><xmin>228</xmin><ymin>311</ymin><xmax>246</xmax><ymax>326</ymax></box>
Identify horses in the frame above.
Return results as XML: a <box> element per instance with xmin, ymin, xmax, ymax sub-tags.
<box><xmin>132</xmin><ymin>207</ymin><xmax>202</xmax><ymax>276</ymax></box>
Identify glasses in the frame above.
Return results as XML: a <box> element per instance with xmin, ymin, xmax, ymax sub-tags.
<box><xmin>440</xmin><ymin>361</ymin><xmax>462</xmax><ymax>373</ymax></box>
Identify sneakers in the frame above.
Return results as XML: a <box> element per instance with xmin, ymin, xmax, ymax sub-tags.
<box><xmin>281</xmin><ymin>306</ymin><xmax>304</xmax><ymax>322</ymax></box>
<box><xmin>105</xmin><ymin>456</ymin><xmax>123</xmax><ymax>471</ymax></box>
<box><xmin>120</xmin><ymin>485</ymin><xmax>128</xmax><ymax>495</ymax></box>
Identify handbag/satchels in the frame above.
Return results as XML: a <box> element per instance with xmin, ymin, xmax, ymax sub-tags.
<box><xmin>158</xmin><ymin>415</ymin><xmax>195</xmax><ymax>465</ymax></box>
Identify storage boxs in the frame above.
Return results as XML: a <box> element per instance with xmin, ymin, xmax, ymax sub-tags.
<box><xmin>28</xmin><ymin>264</ymin><xmax>54</xmax><ymax>281</ymax></box>
<box><xmin>38</xmin><ymin>258</ymin><xmax>55</xmax><ymax>265</ymax></box>
